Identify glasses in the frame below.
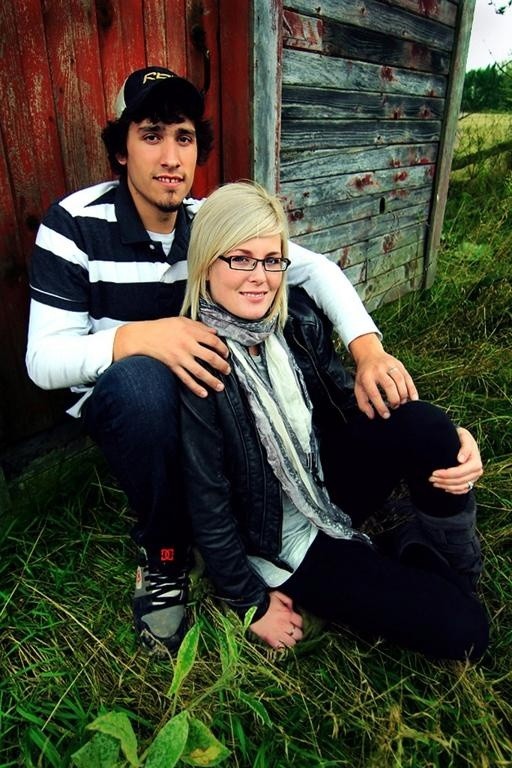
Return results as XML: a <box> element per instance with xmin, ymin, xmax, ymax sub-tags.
<box><xmin>217</xmin><ymin>253</ymin><xmax>292</xmax><ymax>273</ymax></box>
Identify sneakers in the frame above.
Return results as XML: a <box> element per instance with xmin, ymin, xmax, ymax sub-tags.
<box><xmin>130</xmin><ymin>540</ymin><xmax>196</xmax><ymax>667</ymax></box>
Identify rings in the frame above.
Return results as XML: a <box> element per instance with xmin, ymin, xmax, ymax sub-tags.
<box><xmin>287</xmin><ymin>624</ymin><xmax>296</xmax><ymax>637</ymax></box>
<box><xmin>386</xmin><ymin>366</ymin><xmax>400</xmax><ymax>376</ymax></box>
<box><xmin>465</xmin><ymin>481</ymin><xmax>475</xmax><ymax>493</ymax></box>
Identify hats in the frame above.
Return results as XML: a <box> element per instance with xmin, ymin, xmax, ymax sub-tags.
<box><xmin>113</xmin><ymin>64</ymin><xmax>205</xmax><ymax>125</ymax></box>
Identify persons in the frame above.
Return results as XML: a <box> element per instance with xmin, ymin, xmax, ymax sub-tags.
<box><xmin>22</xmin><ymin>61</ymin><xmax>420</xmax><ymax>673</ymax></box>
<box><xmin>156</xmin><ymin>176</ymin><xmax>494</xmax><ymax>668</ymax></box>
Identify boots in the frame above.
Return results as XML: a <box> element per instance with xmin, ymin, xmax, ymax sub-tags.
<box><xmin>383</xmin><ymin>493</ymin><xmax>484</xmax><ymax>576</ymax></box>
